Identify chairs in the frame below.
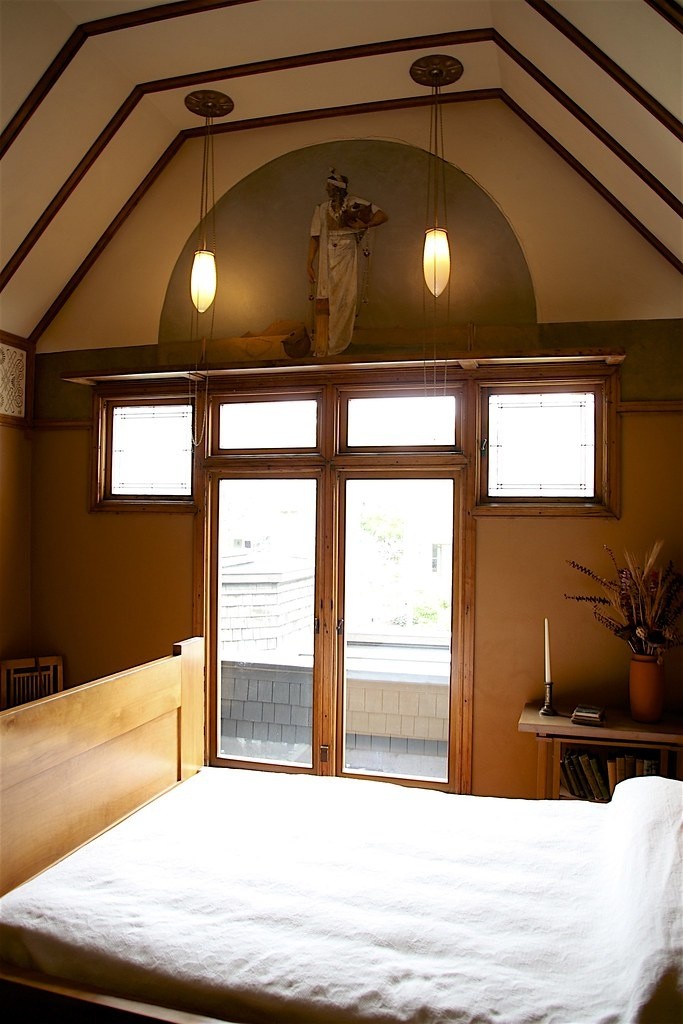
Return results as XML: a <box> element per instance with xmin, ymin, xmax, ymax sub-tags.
<box><xmin>0</xmin><ymin>656</ymin><xmax>63</xmax><ymax>712</ymax></box>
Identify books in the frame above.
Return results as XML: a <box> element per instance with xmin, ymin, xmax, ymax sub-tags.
<box><xmin>571</xmin><ymin>703</ymin><xmax>608</xmax><ymax>727</ymax></box>
<box><xmin>560</xmin><ymin>748</ymin><xmax>660</xmax><ymax>800</ymax></box>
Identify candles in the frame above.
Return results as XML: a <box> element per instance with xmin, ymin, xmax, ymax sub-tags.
<box><xmin>545</xmin><ymin>618</ymin><xmax>551</xmax><ymax>682</ymax></box>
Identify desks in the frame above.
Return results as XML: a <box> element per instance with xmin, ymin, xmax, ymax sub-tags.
<box><xmin>518</xmin><ymin>702</ymin><xmax>683</xmax><ymax>800</ymax></box>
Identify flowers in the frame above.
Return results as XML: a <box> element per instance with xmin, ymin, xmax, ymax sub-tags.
<box><xmin>562</xmin><ymin>544</ymin><xmax>683</xmax><ymax>665</ymax></box>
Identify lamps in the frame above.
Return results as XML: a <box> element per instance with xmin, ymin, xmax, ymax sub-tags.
<box><xmin>409</xmin><ymin>54</ymin><xmax>464</xmax><ymax>400</ymax></box>
<box><xmin>183</xmin><ymin>89</ymin><xmax>234</xmax><ymax>448</ymax></box>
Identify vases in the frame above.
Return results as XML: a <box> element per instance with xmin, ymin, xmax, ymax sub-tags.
<box><xmin>630</xmin><ymin>654</ymin><xmax>663</xmax><ymax>723</ymax></box>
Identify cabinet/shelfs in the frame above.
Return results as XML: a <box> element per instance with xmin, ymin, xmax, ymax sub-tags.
<box><xmin>0</xmin><ymin>637</ymin><xmax>683</xmax><ymax>1024</ymax></box>
